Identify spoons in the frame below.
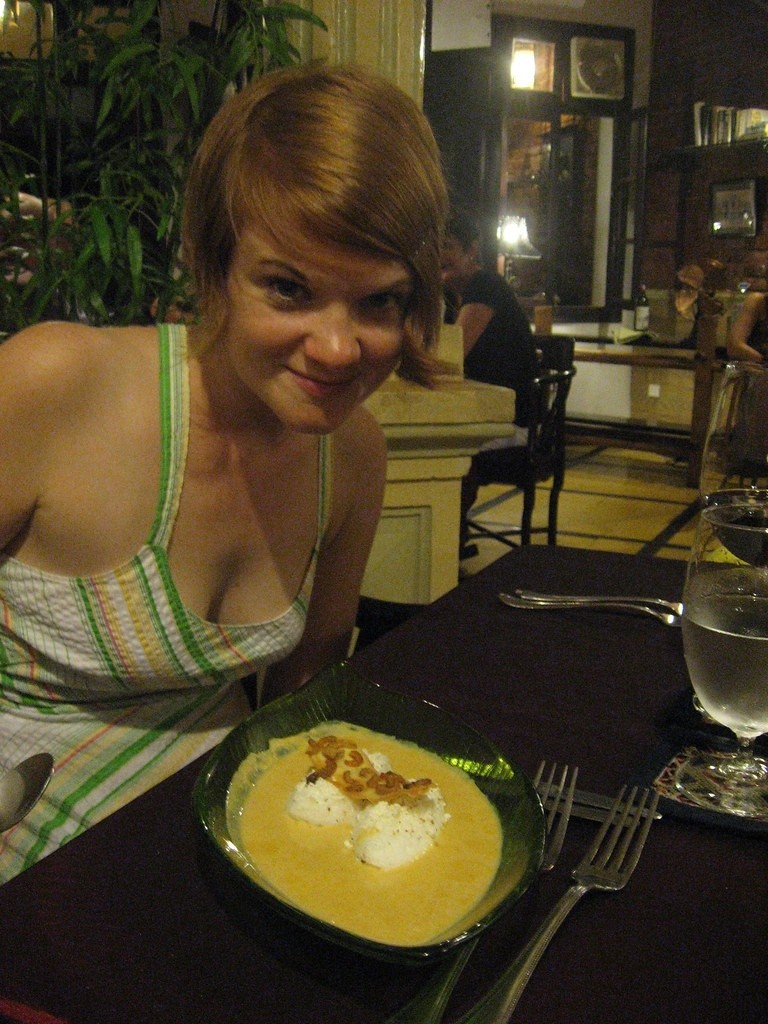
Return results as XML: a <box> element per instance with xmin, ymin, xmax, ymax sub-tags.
<box><xmin>0</xmin><ymin>753</ymin><xmax>54</xmax><ymax>833</ymax></box>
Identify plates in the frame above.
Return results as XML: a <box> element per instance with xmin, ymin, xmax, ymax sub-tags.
<box><xmin>191</xmin><ymin>657</ymin><xmax>547</xmax><ymax>959</ymax></box>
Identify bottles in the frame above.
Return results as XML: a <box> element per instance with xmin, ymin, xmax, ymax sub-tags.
<box><xmin>633</xmin><ymin>285</ymin><xmax>651</xmax><ymax>331</ymax></box>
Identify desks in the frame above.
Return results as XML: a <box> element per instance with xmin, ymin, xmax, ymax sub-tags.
<box><xmin>360</xmin><ymin>375</ymin><xmax>519</xmax><ymax>603</ymax></box>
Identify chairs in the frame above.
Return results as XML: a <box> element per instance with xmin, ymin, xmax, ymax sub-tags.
<box><xmin>481</xmin><ymin>335</ymin><xmax>578</xmax><ymax>545</ymax></box>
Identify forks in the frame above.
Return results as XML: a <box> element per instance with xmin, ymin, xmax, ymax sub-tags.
<box><xmin>421</xmin><ymin>763</ymin><xmax>580</xmax><ymax>1024</ymax></box>
<box><xmin>473</xmin><ymin>784</ymin><xmax>660</xmax><ymax>1024</ymax></box>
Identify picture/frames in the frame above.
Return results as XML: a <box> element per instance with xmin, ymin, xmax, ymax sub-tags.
<box><xmin>708</xmin><ymin>177</ymin><xmax>758</xmax><ymax>238</ymax></box>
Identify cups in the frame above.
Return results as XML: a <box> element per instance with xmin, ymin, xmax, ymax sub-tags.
<box><xmin>534</xmin><ymin>306</ymin><xmax>552</xmax><ymax>337</ymax></box>
<box><xmin>717</xmin><ymin>110</ymin><xmax>732</xmax><ymax>143</ymax></box>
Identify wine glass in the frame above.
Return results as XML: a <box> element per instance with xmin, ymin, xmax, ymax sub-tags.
<box><xmin>700</xmin><ymin>360</ymin><xmax>768</xmax><ymax>505</ymax></box>
<box><xmin>674</xmin><ymin>505</ymin><xmax>768</xmax><ymax>817</ymax></box>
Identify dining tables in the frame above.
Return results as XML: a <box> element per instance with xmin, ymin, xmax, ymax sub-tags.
<box><xmin>0</xmin><ymin>543</ymin><xmax>768</xmax><ymax>1024</ymax></box>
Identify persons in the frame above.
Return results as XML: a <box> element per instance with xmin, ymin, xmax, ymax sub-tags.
<box><xmin>728</xmin><ymin>292</ymin><xmax>768</xmax><ymax>435</ymax></box>
<box><xmin>440</xmin><ymin>210</ymin><xmax>543</xmax><ymax>561</ymax></box>
<box><xmin>0</xmin><ymin>66</ymin><xmax>448</xmax><ymax>885</ymax></box>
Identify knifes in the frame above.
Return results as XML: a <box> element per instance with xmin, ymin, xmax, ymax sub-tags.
<box><xmin>532</xmin><ymin>780</ymin><xmax>662</xmax><ymax>819</ymax></box>
<box><xmin>545</xmin><ymin>800</ymin><xmax>639</xmax><ymax>829</ymax></box>
